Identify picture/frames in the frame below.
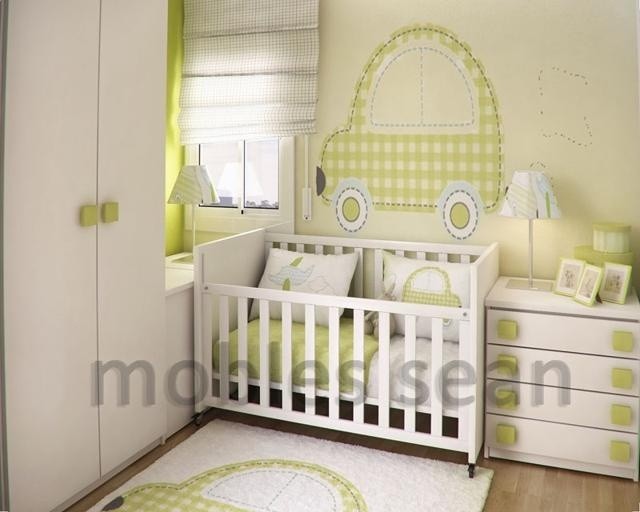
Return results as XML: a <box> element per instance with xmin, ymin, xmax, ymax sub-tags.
<box><xmin>554</xmin><ymin>258</ymin><xmax>585</xmax><ymax>296</ymax></box>
<box><xmin>572</xmin><ymin>263</ymin><xmax>601</xmax><ymax>306</ymax></box>
<box><xmin>599</xmin><ymin>262</ymin><xmax>629</xmax><ymax>303</ymax></box>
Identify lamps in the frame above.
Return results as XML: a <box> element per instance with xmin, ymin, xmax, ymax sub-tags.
<box><xmin>167</xmin><ymin>166</ymin><xmax>220</xmax><ymax>264</ymax></box>
<box><xmin>499</xmin><ymin>170</ymin><xmax>562</xmax><ymax>293</ymax></box>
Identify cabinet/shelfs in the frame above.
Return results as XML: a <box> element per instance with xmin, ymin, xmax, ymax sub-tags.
<box><xmin>0</xmin><ymin>0</ymin><xmax>168</xmax><ymax>512</ymax></box>
<box><xmin>483</xmin><ymin>276</ymin><xmax>640</xmax><ymax>482</ymax></box>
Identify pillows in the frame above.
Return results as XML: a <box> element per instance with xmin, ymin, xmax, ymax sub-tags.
<box><xmin>382</xmin><ymin>250</ymin><xmax>475</xmax><ymax>341</ymax></box>
<box><xmin>248</xmin><ymin>248</ymin><xmax>357</xmax><ymax>327</ymax></box>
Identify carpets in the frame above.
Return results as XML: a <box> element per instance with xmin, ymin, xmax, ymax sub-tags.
<box><xmin>87</xmin><ymin>417</ymin><xmax>495</xmax><ymax>511</ymax></box>
